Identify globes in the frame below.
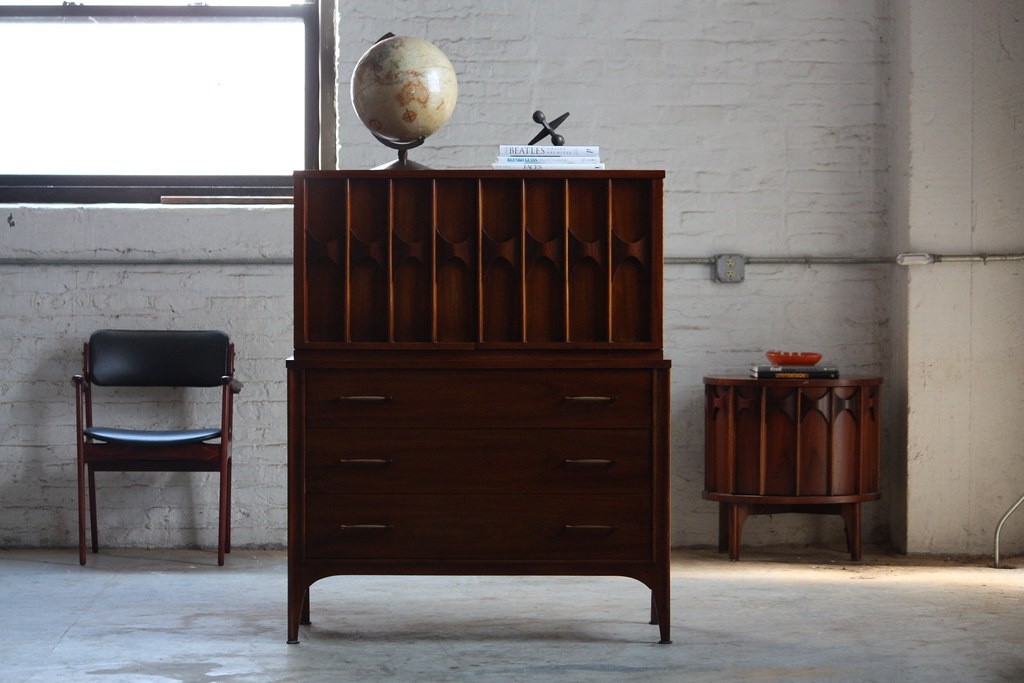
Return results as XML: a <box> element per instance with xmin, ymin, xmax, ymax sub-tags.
<box><xmin>350</xmin><ymin>32</ymin><xmax>459</xmax><ymax>171</ymax></box>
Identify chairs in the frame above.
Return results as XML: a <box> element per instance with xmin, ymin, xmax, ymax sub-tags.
<box><xmin>72</xmin><ymin>327</ymin><xmax>244</xmax><ymax>565</ymax></box>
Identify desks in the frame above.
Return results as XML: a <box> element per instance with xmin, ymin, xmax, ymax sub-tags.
<box><xmin>702</xmin><ymin>374</ymin><xmax>886</xmax><ymax>560</ymax></box>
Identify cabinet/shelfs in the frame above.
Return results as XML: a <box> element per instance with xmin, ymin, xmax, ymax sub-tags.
<box><xmin>284</xmin><ymin>167</ymin><xmax>673</xmax><ymax>651</ymax></box>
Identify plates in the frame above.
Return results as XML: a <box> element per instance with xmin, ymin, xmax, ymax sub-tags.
<box><xmin>768</xmin><ymin>351</ymin><xmax>821</xmax><ymax>366</ymax></box>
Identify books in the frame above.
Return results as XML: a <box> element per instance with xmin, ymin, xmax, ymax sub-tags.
<box><xmin>490</xmin><ymin>145</ymin><xmax>605</xmax><ymax>170</ymax></box>
<box><xmin>749</xmin><ymin>363</ymin><xmax>840</xmax><ymax>380</ymax></box>
